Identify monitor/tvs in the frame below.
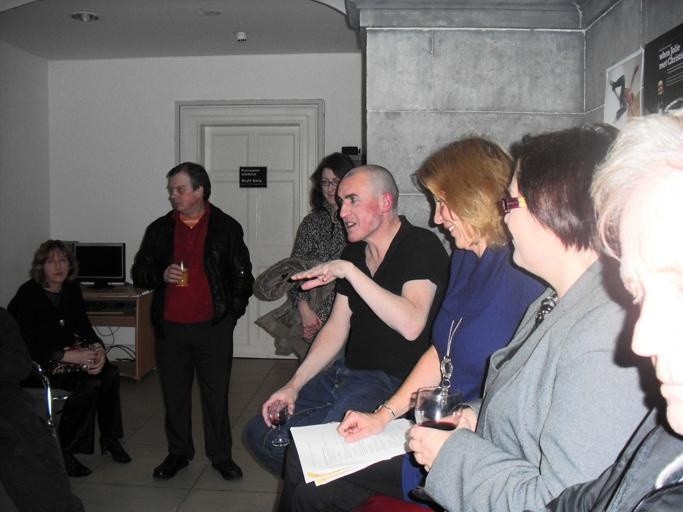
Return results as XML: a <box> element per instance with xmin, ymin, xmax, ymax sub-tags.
<box><xmin>74</xmin><ymin>242</ymin><xmax>126</xmax><ymax>291</ymax></box>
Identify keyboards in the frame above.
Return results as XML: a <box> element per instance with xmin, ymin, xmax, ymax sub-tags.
<box><xmin>84</xmin><ymin>302</ymin><xmax>129</xmax><ymax>314</ymax></box>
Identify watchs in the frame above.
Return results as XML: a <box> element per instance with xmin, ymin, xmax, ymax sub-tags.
<box><xmin>373</xmin><ymin>403</ymin><xmax>397</xmax><ymax>420</ymax></box>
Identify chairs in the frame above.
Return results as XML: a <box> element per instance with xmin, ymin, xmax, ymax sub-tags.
<box><xmin>18</xmin><ymin>357</ymin><xmax>87</xmax><ymax>467</ymax></box>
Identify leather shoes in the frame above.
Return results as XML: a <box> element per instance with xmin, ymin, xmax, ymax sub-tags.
<box><xmin>100</xmin><ymin>435</ymin><xmax>132</xmax><ymax>463</ymax></box>
<box><xmin>67</xmin><ymin>452</ymin><xmax>91</xmax><ymax>477</ymax></box>
<box><xmin>212</xmin><ymin>460</ymin><xmax>243</xmax><ymax>481</ymax></box>
<box><xmin>153</xmin><ymin>454</ymin><xmax>190</xmax><ymax>480</ymax></box>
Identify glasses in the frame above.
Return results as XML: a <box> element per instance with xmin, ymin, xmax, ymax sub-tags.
<box><xmin>321</xmin><ymin>180</ymin><xmax>340</xmax><ymax>186</ymax></box>
<box><xmin>501</xmin><ymin>196</ymin><xmax>527</xmax><ymax>213</ymax></box>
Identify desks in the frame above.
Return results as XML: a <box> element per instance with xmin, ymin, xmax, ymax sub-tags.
<box><xmin>79</xmin><ymin>284</ymin><xmax>162</xmax><ymax>380</ymax></box>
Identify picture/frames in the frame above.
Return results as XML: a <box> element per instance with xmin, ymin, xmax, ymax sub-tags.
<box><xmin>598</xmin><ymin>48</ymin><xmax>645</xmax><ymax>127</ymax></box>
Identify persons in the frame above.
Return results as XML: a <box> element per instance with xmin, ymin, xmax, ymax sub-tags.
<box><xmin>130</xmin><ymin>161</ymin><xmax>254</xmax><ymax>481</ymax></box>
<box><xmin>273</xmin><ymin>139</ymin><xmax>555</xmax><ymax>512</ymax></box>
<box><xmin>7</xmin><ymin>240</ymin><xmax>134</xmax><ymax>476</ymax></box>
<box><xmin>241</xmin><ymin>165</ymin><xmax>447</xmax><ymax>480</ymax></box>
<box><xmin>406</xmin><ymin>134</ymin><xmax>659</xmax><ymax>512</ymax></box>
<box><xmin>544</xmin><ymin>111</ymin><xmax>683</xmax><ymax>512</ymax></box>
<box><xmin>290</xmin><ymin>152</ymin><xmax>360</xmax><ymax>361</ymax></box>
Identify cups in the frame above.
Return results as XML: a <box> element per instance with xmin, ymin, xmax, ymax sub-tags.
<box><xmin>267</xmin><ymin>402</ymin><xmax>290</xmax><ymax>448</ymax></box>
<box><xmin>175</xmin><ymin>259</ymin><xmax>190</xmax><ymax>288</ymax></box>
<box><xmin>410</xmin><ymin>387</ymin><xmax>463</xmax><ymax>502</ymax></box>
<box><xmin>81</xmin><ymin>345</ymin><xmax>96</xmax><ymax>371</ymax></box>
